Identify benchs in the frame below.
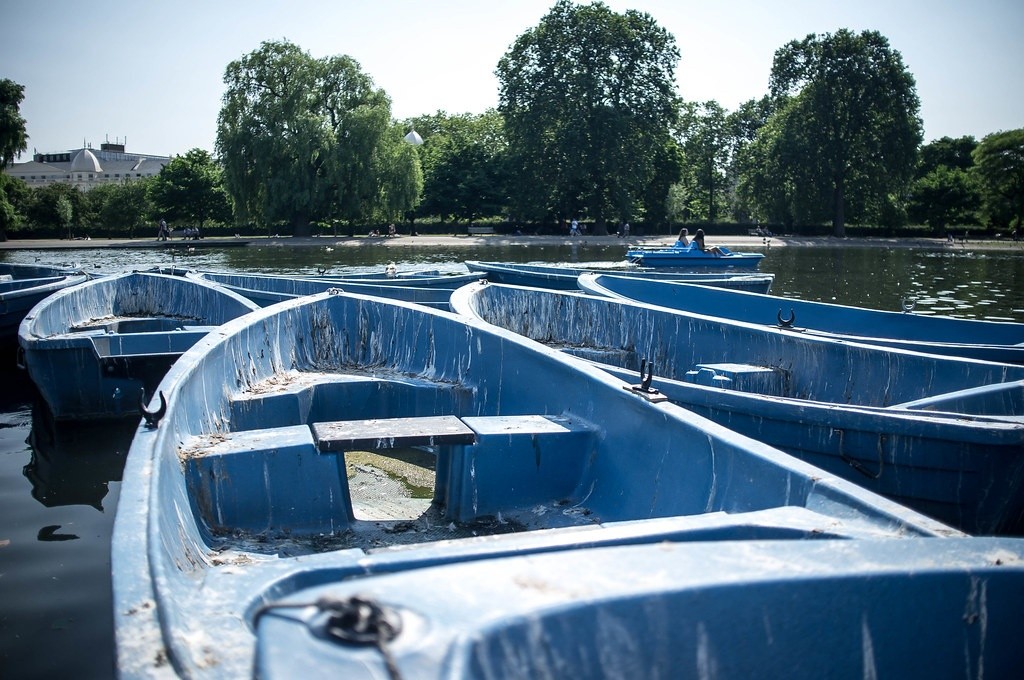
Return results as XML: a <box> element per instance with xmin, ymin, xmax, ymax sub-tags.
<box><xmin>231</xmin><ymin>367</ymin><xmax>477</xmax><ymax>430</ymax></box>
<box><xmin>68</xmin><ymin>314</ymin><xmax>203</xmax><ymax>333</ymax></box>
<box><xmin>468</xmin><ymin>226</ymin><xmax>496</xmax><ymax>236</ymax></box>
<box><xmin>169</xmin><ymin>231</ymin><xmax>188</xmax><ymax>241</ymax></box>
<box><xmin>186</xmin><ymin>416</ymin><xmax>598</xmax><ymax>536</ymax></box>
<box><xmin>63</xmin><ymin>326</ymin><xmax>223</xmax><ymax>357</ymax></box>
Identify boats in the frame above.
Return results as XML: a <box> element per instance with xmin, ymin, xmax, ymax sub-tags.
<box><xmin>0</xmin><ymin>245</ymin><xmax>595</xmax><ymax>359</ymax></box>
<box><xmin>447</xmin><ymin>280</ymin><xmax>1024</xmax><ymax>535</ymax></box>
<box><xmin>20</xmin><ymin>270</ymin><xmax>264</xmax><ymax>426</ymax></box>
<box><xmin>625</xmin><ymin>248</ymin><xmax>764</xmax><ymax>271</ymax></box>
<box><xmin>111</xmin><ymin>285</ymin><xmax>1024</xmax><ymax>679</ymax></box>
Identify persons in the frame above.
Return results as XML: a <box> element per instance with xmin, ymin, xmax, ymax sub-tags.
<box><xmin>679</xmin><ymin>228</ymin><xmax>689</xmax><ymax>245</ymax></box>
<box><xmin>389</xmin><ymin>224</ymin><xmax>396</xmax><ymax>237</ymax></box>
<box><xmin>756</xmin><ymin>225</ymin><xmax>769</xmax><ymax>237</ymax></box>
<box><xmin>1012</xmin><ymin>228</ymin><xmax>1017</xmax><ymax>241</ymax></box>
<box><xmin>948</xmin><ymin>230</ymin><xmax>954</xmax><ymax>241</ymax></box>
<box><xmin>693</xmin><ymin>229</ymin><xmax>728</xmax><ymax>256</ymax></box>
<box><xmin>617</xmin><ymin>220</ymin><xmax>630</xmax><ymax>239</ymax></box>
<box><xmin>184</xmin><ymin>226</ymin><xmax>199</xmax><ymax>239</ymax></box>
<box><xmin>965</xmin><ymin>230</ymin><xmax>969</xmax><ymax>238</ymax></box>
<box><xmin>369</xmin><ymin>230</ymin><xmax>379</xmax><ymax>237</ymax></box>
<box><xmin>156</xmin><ymin>218</ymin><xmax>167</xmax><ymax>241</ymax></box>
<box><xmin>571</xmin><ymin>218</ymin><xmax>581</xmax><ymax>236</ymax></box>
